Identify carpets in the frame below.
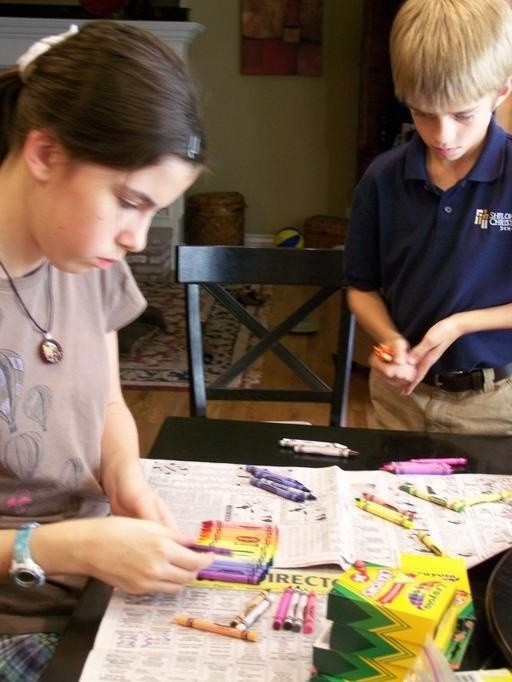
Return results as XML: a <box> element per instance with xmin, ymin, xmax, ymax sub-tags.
<box><xmin>111</xmin><ymin>271</ymin><xmax>272</xmax><ymax>391</ymax></box>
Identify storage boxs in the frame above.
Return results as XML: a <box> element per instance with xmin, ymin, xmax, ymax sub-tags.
<box><xmin>304</xmin><ymin>214</ymin><xmax>351</xmax><ymax>248</ymax></box>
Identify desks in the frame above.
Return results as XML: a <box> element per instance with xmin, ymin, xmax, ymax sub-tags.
<box><xmin>36</xmin><ymin>416</ymin><xmax>512</xmax><ymax>682</ymax></box>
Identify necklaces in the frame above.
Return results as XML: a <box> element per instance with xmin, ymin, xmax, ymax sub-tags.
<box><xmin>0</xmin><ymin>256</ymin><xmax>65</xmax><ymax>363</ymax></box>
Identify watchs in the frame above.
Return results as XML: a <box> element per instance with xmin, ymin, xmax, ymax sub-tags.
<box><xmin>8</xmin><ymin>519</ymin><xmax>47</xmax><ymax>595</ymax></box>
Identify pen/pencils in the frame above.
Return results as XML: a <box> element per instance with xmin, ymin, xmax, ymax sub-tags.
<box><xmin>247</xmin><ymin>465</ymin><xmax>317</xmax><ymax>502</ymax></box>
<box><xmin>369</xmin><ymin>342</ymin><xmax>397</xmax><ymax>364</ymax></box>
<box><xmin>278</xmin><ymin>438</ymin><xmax>361</xmax><ymax>458</ymax></box>
<box><xmin>185</xmin><ymin>520</ymin><xmax>278</xmax><ymax>586</ymax></box>
<box><xmin>175</xmin><ymin>615</ymin><xmax>257</xmax><ymax>642</ymax></box>
<box><xmin>274</xmin><ymin>586</ymin><xmax>316</xmax><ymax>634</ymax></box>
<box><xmin>353</xmin><ymin>485</ymin><xmax>512</xmax><ymax>556</ymax></box>
<box><xmin>378</xmin><ymin>457</ymin><xmax>469</xmax><ymax>474</ymax></box>
<box><xmin>230</xmin><ymin>588</ymin><xmax>276</xmax><ymax>632</ymax></box>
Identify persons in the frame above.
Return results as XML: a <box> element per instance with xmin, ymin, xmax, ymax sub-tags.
<box><xmin>342</xmin><ymin>0</ymin><xmax>512</xmax><ymax>437</ymax></box>
<box><xmin>0</xmin><ymin>21</ymin><xmax>217</xmax><ymax>682</ymax></box>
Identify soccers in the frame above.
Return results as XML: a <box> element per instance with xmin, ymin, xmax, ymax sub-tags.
<box><xmin>274</xmin><ymin>226</ymin><xmax>305</xmax><ymax>249</ymax></box>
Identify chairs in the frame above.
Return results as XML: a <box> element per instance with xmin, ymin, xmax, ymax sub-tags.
<box><xmin>175</xmin><ymin>246</ymin><xmax>356</xmax><ymax>428</ymax></box>
